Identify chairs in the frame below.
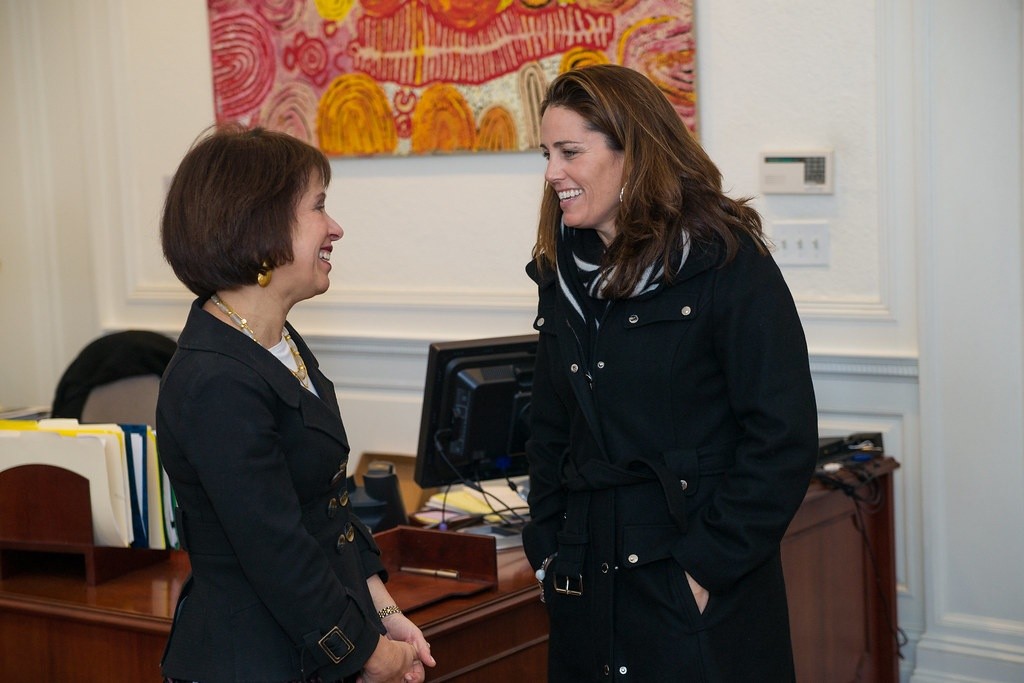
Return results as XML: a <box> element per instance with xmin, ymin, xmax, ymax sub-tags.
<box><xmin>51</xmin><ymin>329</ymin><xmax>183</xmax><ymax>431</ymax></box>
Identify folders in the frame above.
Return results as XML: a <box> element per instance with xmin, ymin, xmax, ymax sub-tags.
<box><xmin>1</xmin><ymin>419</ymin><xmax>181</xmax><ymax>553</ymax></box>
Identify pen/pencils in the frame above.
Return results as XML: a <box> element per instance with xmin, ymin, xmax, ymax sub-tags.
<box><xmin>400</xmin><ymin>565</ymin><xmax>461</xmax><ymax>580</ymax></box>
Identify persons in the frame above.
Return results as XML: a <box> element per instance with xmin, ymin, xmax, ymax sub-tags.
<box><xmin>155</xmin><ymin>124</ymin><xmax>436</xmax><ymax>683</ymax></box>
<box><xmin>522</xmin><ymin>63</ymin><xmax>818</xmax><ymax>683</ymax></box>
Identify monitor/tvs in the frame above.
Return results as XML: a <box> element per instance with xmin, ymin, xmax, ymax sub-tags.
<box><xmin>415</xmin><ymin>333</ymin><xmax>540</xmax><ymax>490</ymax></box>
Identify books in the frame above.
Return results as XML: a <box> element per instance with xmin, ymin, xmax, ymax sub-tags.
<box><xmin>426</xmin><ymin>475</ymin><xmax>530</xmax><ymax>522</ymax></box>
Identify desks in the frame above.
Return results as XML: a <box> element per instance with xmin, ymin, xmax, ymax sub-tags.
<box><xmin>0</xmin><ymin>455</ymin><xmax>901</xmax><ymax>683</ymax></box>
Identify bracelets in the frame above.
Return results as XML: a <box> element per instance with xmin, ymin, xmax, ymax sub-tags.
<box><xmin>535</xmin><ymin>558</ymin><xmax>549</xmax><ymax>603</ymax></box>
<box><xmin>375</xmin><ymin>605</ymin><xmax>402</xmax><ymax>619</ymax></box>
